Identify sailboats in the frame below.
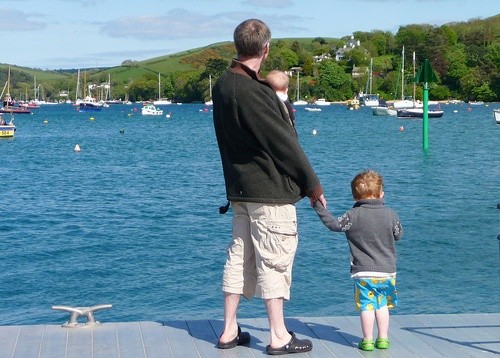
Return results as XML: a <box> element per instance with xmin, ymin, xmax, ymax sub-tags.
<box><xmin>153</xmin><ymin>73</ymin><xmax>171</xmax><ymax>104</ymax></box>
<box><xmin>0</xmin><ymin>64</ymin><xmax>131</xmax><ymax>138</ymax></box>
<box><xmin>205</xmin><ymin>75</ymin><xmax>213</xmax><ymax>105</ymax></box>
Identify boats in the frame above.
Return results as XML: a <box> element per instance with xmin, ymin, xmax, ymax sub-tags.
<box><xmin>398</xmin><ymin>47</ymin><xmax>445</xmax><ymax>118</ymax></box>
<box><xmin>293</xmin><ymin>46</ymin><xmax>484</xmax><ymax>117</ymax></box>
<box><xmin>142</xmin><ymin>105</ymin><xmax>163</xmax><ymax>115</ymax></box>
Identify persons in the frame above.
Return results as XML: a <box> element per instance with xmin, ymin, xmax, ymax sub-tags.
<box><xmin>312</xmin><ymin>169</ymin><xmax>404</xmax><ymax>352</ymax></box>
<box><xmin>211</xmin><ymin>18</ymin><xmax>326</xmax><ymax>354</ymax></box>
<box><xmin>266</xmin><ymin>70</ymin><xmax>296</xmax><ymax>125</ymax></box>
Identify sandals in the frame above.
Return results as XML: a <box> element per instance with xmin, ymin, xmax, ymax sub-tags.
<box><xmin>358</xmin><ymin>338</ymin><xmax>374</xmax><ymax>350</ymax></box>
<box><xmin>376</xmin><ymin>338</ymin><xmax>390</xmax><ymax>349</ymax></box>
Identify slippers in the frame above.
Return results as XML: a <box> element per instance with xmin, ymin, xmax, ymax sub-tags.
<box><xmin>265</xmin><ymin>331</ymin><xmax>313</xmax><ymax>356</ymax></box>
<box><xmin>218</xmin><ymin>325</ymin><xmax>250</xmax><ymax>349</ymax></box>
